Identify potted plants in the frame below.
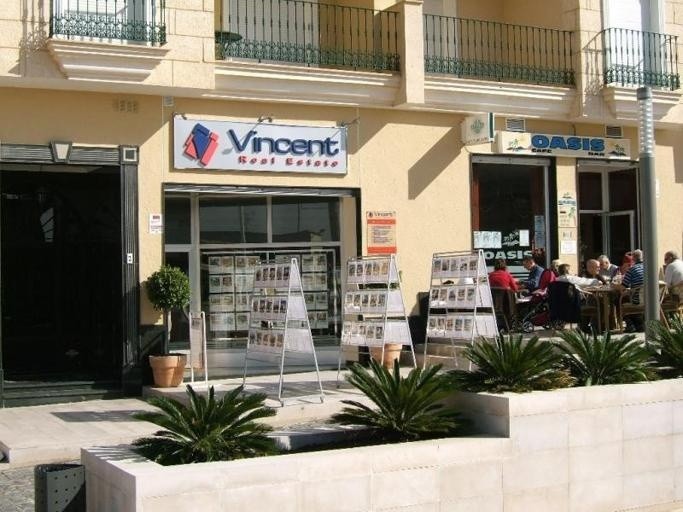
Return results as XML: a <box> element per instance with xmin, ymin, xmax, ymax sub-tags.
<box><xmin>363</xmin><ymin>268</ymin><xmax>404</xmax><ymax>369</ymax></box>
<box><xmin>141</xmin><ymin>263</ymin><xmax>189</xmax><ymax>386</ymax></box>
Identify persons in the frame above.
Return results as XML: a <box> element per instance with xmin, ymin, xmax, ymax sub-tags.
<box><xmin>247</xmin><ymin>265</ymin><xmax>288</xmax><ymax>348</ymax></box>
<box><xmin>488</xmin><ymin>258</ymin><xmax>521</xmax><ymax>290</ymax></box>
<box><xmin>439</xmin><ymin>289</ymin><xmax>446</xmax><ymax>301</ymax></box>
<box><xmin>517</xmin><ymin>256</ymin><xmax>544</xmax><ymax>291</ymax></box>
<box><xmin>550</xmin><ymin>248</ymin><xmax>646</xmax><ymax>332</ymax></box>
<box><xmin>342</xmin><ymin>261</ymin><xmax>389</xmax><ymax>340</ymax></box>
<box><xmin>445</xmin><ymin>319</ymin><xmax>452</xmax><ymax>330</ymax></box>
<box><xmin>210</xmin><ymin>277</ymin><xmax>218</xmax><ymax>287</ymax></box>
<box><xmin>662</xmin><ymin>250</ymin><xmax>682</xmax><ymax>302</ymax></box>
<box><xmin>431</xmin><ymin>289</ymin><xmax>438</xmax><ymax>300</ymax></box>
<box><xmin>437</xmin><ymin>318</ymin><xmax>444</xmax><ymax>329</ymax></box>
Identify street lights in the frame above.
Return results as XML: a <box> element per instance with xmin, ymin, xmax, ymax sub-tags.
<box><xmin>634</xmin><ymin>86</ymin><xmax>664</xmax><ymax>352</ymax></box>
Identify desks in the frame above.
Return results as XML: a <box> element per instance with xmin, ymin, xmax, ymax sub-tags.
<box><xmin>214</xmin><ymin>29</ymin><xmax>241</xmax><ymax>59</ymax></box>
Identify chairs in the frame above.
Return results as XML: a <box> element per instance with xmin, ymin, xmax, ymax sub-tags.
<box><xmin>483</xmin><ymin>266</ymin><xmax>682</xmax><ymax>334</ymax></box>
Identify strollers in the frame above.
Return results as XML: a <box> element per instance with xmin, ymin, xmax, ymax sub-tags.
<box><xmin>521</xmin><ymin>269</ymin><xmax>563</xmax><ymax>333</ymax></box>
